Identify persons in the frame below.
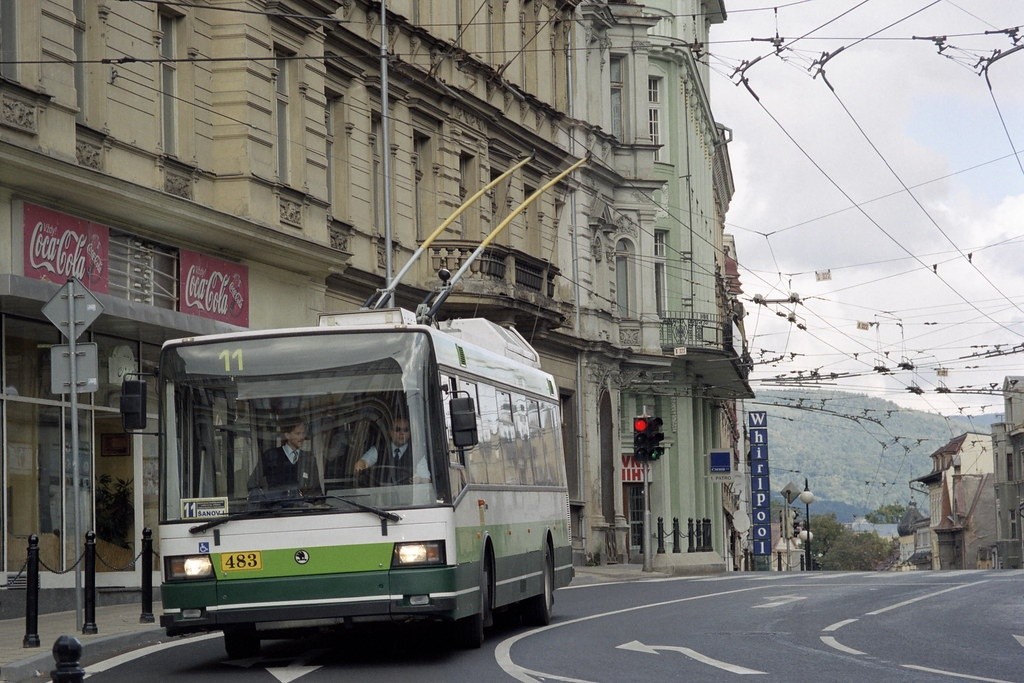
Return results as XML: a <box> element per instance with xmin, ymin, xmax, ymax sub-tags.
<box><xmin>354</xmin><ymin>416</ymin><xmax>431</xmax><ymax>483</ymax></box>
<box><xmin>246</xmin><ymin>416</ymin><xmax>324</xmax><ymax>503</ymax></box>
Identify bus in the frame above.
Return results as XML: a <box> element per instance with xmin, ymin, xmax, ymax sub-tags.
<box><xmin>120</xmin><ymin>148</ymin><xmax>593</xmax><ymax>664</ymax></box>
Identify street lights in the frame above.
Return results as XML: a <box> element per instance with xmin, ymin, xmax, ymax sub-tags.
<box><xmin>800</xmin><ymin>478</ymin><xmax>814</xmax><ymax>571</ymax></box>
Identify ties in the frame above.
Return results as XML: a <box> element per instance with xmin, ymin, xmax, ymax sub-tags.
<box><xmin>394</xmin><ymin>448</ymin><xmax>400</xmax><ymax>474</ymax></box>
<box><xmin>291</xmin><ymin>450</ymin><xmax>298</xmax><ymax>463</ymax></box>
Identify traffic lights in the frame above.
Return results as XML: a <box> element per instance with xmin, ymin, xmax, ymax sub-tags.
<box><xmin>633</xmin><ymin>417</ymin><xmax>649</xmax><ymax>461</ymax></box>
<box><xmin>646</xmin><ymin>416</ymin><xmax>664</xmax><ymax>461</ymax></box>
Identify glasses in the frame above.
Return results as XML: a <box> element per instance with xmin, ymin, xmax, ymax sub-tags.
<box><xmin>392</xmin><ymin>426</ymin><xmax>409</xmax><ymax>432</ymax></box>
